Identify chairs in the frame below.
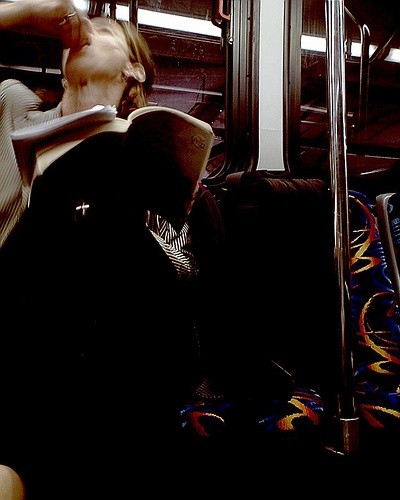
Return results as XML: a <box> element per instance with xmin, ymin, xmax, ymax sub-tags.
<box><xmin>185</xmin><ymin>170</ymin><xmax>399</xmax><ymax>444</ymax></box>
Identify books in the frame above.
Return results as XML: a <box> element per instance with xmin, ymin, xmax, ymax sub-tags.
<box><xmin>26</xmin><ymin>105</ymin><xmax>214</xmax><ymax>235</ymax></box>
<box><xmin>11</xmin><ymin>102</ymin><xmax>117</xmax><ymax>185</ymax></box>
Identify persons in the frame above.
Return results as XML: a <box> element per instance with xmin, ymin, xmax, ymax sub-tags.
<box><xmin>0</xmin><ymin>0</ymin><xmax>203</xmax><ymax>500</ymax></box>
